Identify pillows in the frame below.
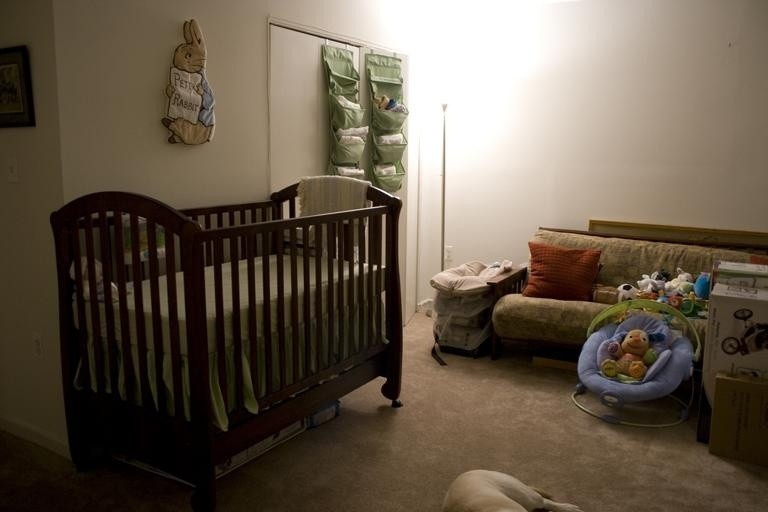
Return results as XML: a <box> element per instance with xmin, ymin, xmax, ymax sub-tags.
<box><xmin>524</xmin><ymin>241</ymin><xmax>601</xmax><ymax>299</ymax></box>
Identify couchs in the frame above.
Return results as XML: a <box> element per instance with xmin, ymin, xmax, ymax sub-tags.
<box><xmin>487</xmin><ymin>221</ymin><xmax>768</xmax><ymax>360</ymax></box>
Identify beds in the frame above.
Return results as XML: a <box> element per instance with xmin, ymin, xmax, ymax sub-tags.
<box><xmin>50</xmin><ymin>176</ymin><xmax>403</xmax><ymax>512</ymax></box>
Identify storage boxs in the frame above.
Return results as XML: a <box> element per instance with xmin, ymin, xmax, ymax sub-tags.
<box><xmin>708</xmin><ymin>371</ymin><xmax>768</xmax><ymax>463</ymax></box>
<box><xmin>703</xmin><ymin>283</ymin><xmax>768</xmax><ymax>407</ymax></box>
<box><xmin>708</xmin><ymin>260</ymin><xmax>768</xmax><ymax>287</ymax></box>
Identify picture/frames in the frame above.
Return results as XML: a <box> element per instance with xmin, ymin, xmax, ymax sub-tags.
<box><xmin>0</xmin><ymin>44</ymin><xmax>36</xmax><ymax>128</ymax></box>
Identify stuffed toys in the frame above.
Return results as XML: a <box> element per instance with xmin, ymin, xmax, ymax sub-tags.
<box><xmin>601</xmin><ymin>329</ymin><xmax>658</xmax><ymax>381</ymax></box>
<box><xmin>617</xmin><ymin>268</ymin><xmax>711</xmax><ymax>319</ymax></box>
